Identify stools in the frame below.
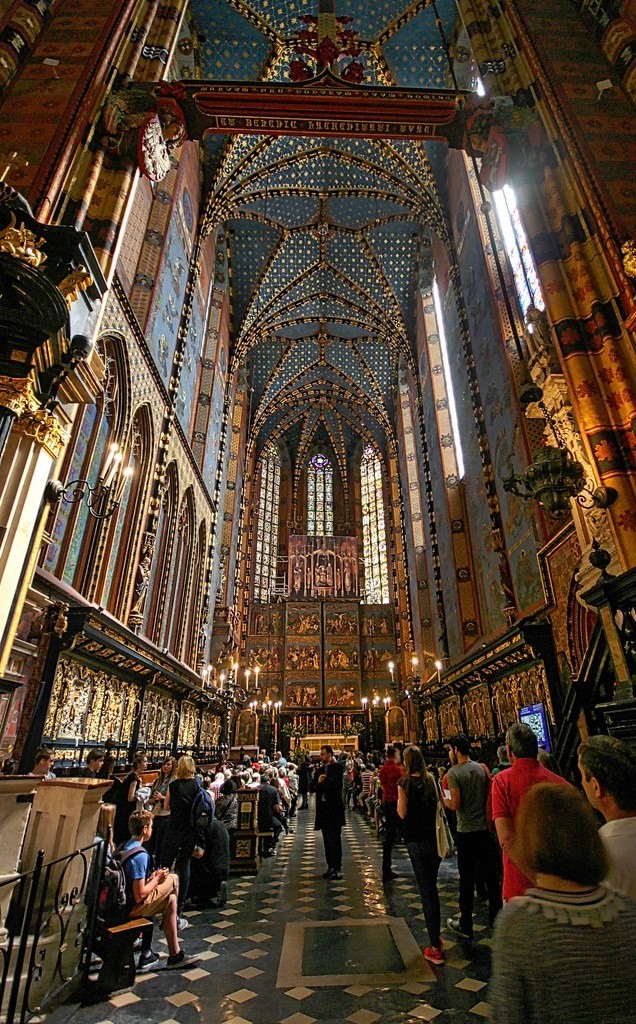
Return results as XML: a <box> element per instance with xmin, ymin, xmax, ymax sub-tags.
<box><xmin>93</xmin><ymin>790</ymin><xmax>286</xmax><ymax>985</ymax></box>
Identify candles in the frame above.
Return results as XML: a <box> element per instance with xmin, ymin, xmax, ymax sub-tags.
<box><xmin>294</xmin><ymin>715</ymin><xmax>350</xmax><ymax>733</ymax></box>
<box><xmin>361</xmin><ymin>657</ymin><xmax>441</xmax><ymax>722</ymax></box>
<box><xmin>98</xmin><ymin>442</ymin><xmax>134</xmax><ymax>505</ymax></box>
<box><xmin>202</xmin><ymin>663</ymin><xmax>282</xmax><ymax>741</ymax></box>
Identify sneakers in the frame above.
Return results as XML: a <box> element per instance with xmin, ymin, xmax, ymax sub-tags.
<box><xmin>424</xmin><ymin>946</ymin><xmax>444</xmax><ymax>964</ymax></box>
<box><xmin>168</xmin><ymin>949</ymin><xmax>202</xmax><ymax>969</ymax></box>
<box><xmin>139</xmin><ymin>948</ymin><xmax>159</xmax><ymax>968</ymax></box>
<box><xmin>447</xmin><ymin>918</ymin><xmax>474</xmax><ymax>937</ymax></box>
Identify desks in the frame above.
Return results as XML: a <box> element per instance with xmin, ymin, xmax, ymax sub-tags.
<box><xmin>290</xmin><ymin>734</ymin><xmax>359</xmax><ymax>763</ymax></box>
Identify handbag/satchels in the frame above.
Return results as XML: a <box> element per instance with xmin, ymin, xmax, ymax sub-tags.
<box><xmin>429</xmin><ymin>773</ymin><xmax>455</xmax><ymax>859</ymax></box>
<box><xmin>481</xmin><ymin>762</ymin><xmax>495</xmax><ymax>832</ymax></box>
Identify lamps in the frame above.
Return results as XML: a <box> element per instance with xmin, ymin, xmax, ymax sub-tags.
<box><xmin>503</xmin><ymin>360</ymin><xmax>587</xmax><ymax>518</ymax></box>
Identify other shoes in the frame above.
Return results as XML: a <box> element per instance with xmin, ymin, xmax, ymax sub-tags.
<box><xmin>217</xmin><ymin>882</ymin><xmax>228</xmax><ymax>906</ymax></box>
<box><xmin>159</xmin><ymin>916</ymin><xmax>188</xmax><ymax>932</ymax></box>
<box><xmin>367</xmin><ymin>811</ymin><xmax>372</xmax><ymax>817</ymax></box>
<box><xmin>323</xmin><ymin>870</ymin><xmax>341</xmax><ymax>881</ymax></box>
<box><xmin>286</xmin><ymin>829</ymin><xmax>293</xmax><ymax>835</ymax></box>
<box><xmin>133</xmin><ymin>937</ymin><xmax>143</xmax><ymax>948</ymax></box>
<box><xmin>268</xmin><ymin>848</ymin><xmax>278</xmax><ymax>856</ymax></box>
<box><xmin>287</xmin><ymin>813</ymin><xmax>296</xmax><ymax>817</ymax></box>
<box><xmin>298</xmin><ymin>804</ymin><xmax>308</xmax><ymax>810</ymax></box>
<box><xmin>382</xmin><ymin>872</ymin><xmax>401</xmax><ymax>879</ymax></box>
<box><xmin>379</xmin><ymin>825</ymin><xmax>384</xmax><ymax>832</ymax></box>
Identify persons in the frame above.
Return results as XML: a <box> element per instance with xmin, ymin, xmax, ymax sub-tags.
<box><xmin>487</xmin><ymin>722</ymin><xmax>590</xmax><ymax>907</ymax></box>
<box><xmin>28</xmin><ymin>747</ymin><xmax>56</xmax><ymax>781</ymax></box>
<box><xmin>84</xmin><ymin>802</ymin><xmax>143</xmax><ymax>953</ymax></box>
<box><xmin>397</xmin><ymin>746</ymin><xmax>448</xmax><ymax>965</ymax></box>
<box><xmin>576</xmin><ymin>736</ymin><xmax>636</xmax><ymax>904</ymax></box>
<box><xmin>66</xmin><ymin>749</ymin><xmax>105</xmax><ymax>778</ymax></box>
<box><xmin>113</xmin><ymin>753</ymin><xmax>148</xmax><ymax>847</ymax></box>
<box><xmin>465</xmin><ymin>109</ymin><xmax>511</xmax><ymax>192</ymax></box>
<box><xmin>442</xmin><ymin>734</ymin><xmax>503</xmax><ymax>938</ymax></box>
<box><xmin>115</xmin><ymin>809</ymin><xmax>202</xmax><ymax>969</ymax></box>
<box><xmin>110</xmin><ymin>110</ymin><xmax>186</xmax><ymax>182</ymax></box>
<box><xmin>100</xmin><ymin>742</ymin><xmax>559</xmax><ymax>915</ymax></box>
<box><xmin>378</xmin><ymin>746</ymin><xmax>405</xmax><ymax>879</ymax></box>
<box><xmin>154</xmin><ymin>756</ymin><xmax>204</xmax><ymax>931</ymax></box>
<box><xmin>309</xmin><ymin>745</ymin><xmax>346</xmax><ymax>879</ymax></box>
<box><xmin>255</xmin><ymin>774</ymin><xmax>282</xmax><ymax>856</ymax></box>
<box><xmin>491</xmin><ymin>782</ymin><xmax>636</xmax><ymax>1024</ymax></box>
<box><xmin>200</xmin><ymin>816</ymin><xmax>230</xmax><ymax>908</ymax></box>
<box><xmin>294</xmin><ymin>753</ymin><xmax>310</xmax><ymax>810</ymax></box>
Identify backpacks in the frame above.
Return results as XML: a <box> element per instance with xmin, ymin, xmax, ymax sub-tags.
<box><xmin>191</xmin><ymin>785</ymin><xmax>213</xmax><ymax>827</ymax></box>
<box><xmin>97</xmin><ymin>843</ymin><xmax>149</xmax><ymax>923</ymax></box>
